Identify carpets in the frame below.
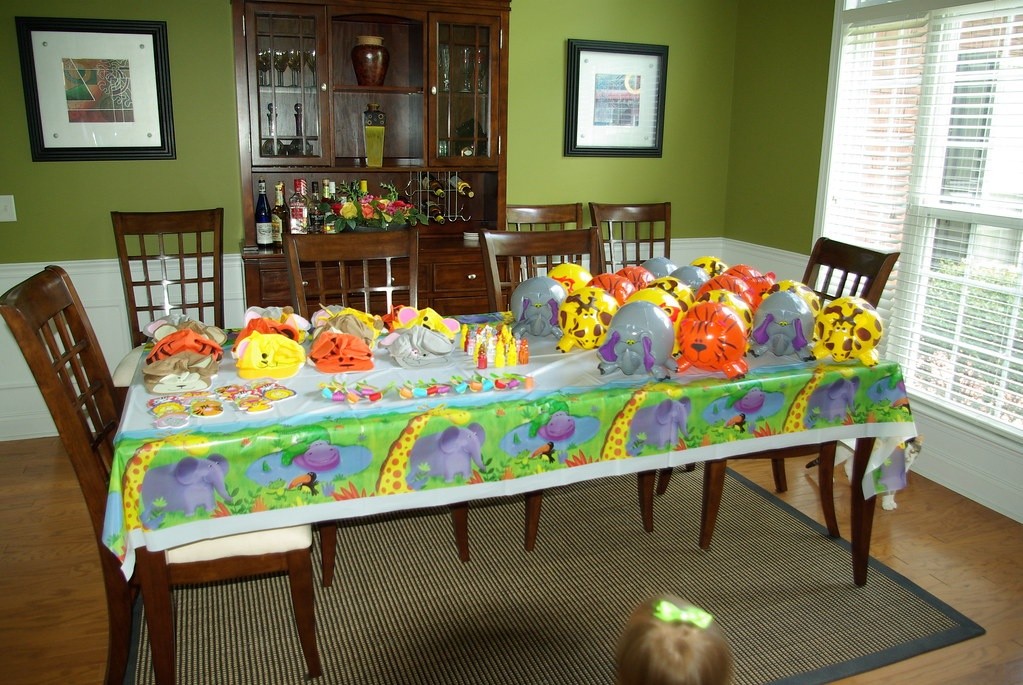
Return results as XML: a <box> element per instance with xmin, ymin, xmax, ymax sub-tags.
<box><xmin>125</xmin><ymin>461</ymin><xmax>985</xmax><ymax>685</ymax></box>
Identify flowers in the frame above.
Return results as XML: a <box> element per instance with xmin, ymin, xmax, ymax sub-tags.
<box><xmin>298</xmin><ymin>179</ymin><xmax>429</xmax><ymax>233</ymax></box>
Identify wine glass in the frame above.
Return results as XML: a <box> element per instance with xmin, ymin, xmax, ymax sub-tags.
<box><xmin>439</xmin><ymin>45</ymin><xmax>487</xmax><ymax>93</ymax></box>
<box><xmin>288</xmin><ymin>48</ymin><xmax>317</xmax><ymax>87</ymax></box>
<box><xmin>258</xmin><ymin>48</ymin><xmax>288</xmax><ymax>86</ymax></box>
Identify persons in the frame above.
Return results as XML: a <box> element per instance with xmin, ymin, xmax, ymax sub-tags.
<box><xmin>614</xmin><ymin>594</ymin><xmax>734</xmax><ymax>685</ymax></box>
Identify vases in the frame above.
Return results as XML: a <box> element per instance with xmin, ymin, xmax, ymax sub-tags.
<box><xmin>344</xmin><ymin>221</ymin><xmax>408</xmax><ymax>265</ymax></box>
<box><xmin>364</xmin><ymin>104</ymin><xmax>385</xmax><ymax>168</ymax></box>
<box><xmin>352</xmin><ymin>36</ymin><xmax>390</xmax><ymax>87</ymax></box>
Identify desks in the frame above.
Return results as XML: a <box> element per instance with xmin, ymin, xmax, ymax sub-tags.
<box><xmin>102</xmin><ymin>311</ymin><xmax>923</xmax><ymax>685</ymax></box>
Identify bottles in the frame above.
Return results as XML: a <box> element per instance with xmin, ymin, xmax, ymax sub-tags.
<box><xmin>421</xmin><ymin>174</ymin><xmax>445</xmax><ymax>198</ymax></box>
<box><xmin>321</xmin><ymin>180</ymin><xmax>369</xmax><ymax>234</ymax></box>
<box><xmin>308</xmin><ymin>181</ymin><xmax>323</xmax><ymax>233</ymax></box>
<box><xmin>447</xmin><ymin>174</ymin><xmax>474</xmax><ymax>198</ymax></box>
<box><xmin>272</xmin><ymin>181</ymin><xmax>289</xmax><ymax>250</ymax></box>
<box><xmin>439</xmin><ymin>138</ymin><xmax>448</xmax><ymax>156</ymax></box>
<box><xmin>362</xmin><ymin>104</ymin><xmax>386</xmax><ymax>127</ymax></box>
<box><xmin>421</xmin><ymin>199</ymin><xmax>445</xmax><ymax>224</ymax></box>
<box><xmin>288</xmin><ymin>103</ymin><xmax>312</xmax><ymax>157</ymax></box>
<box><xmin>290</xmin><ymin>179</ymin><xmax>310</xmax><ymax>234</ymax></box>
<box><xmin>352</xmin><ymin>36</ymin><xmax>388</xmax><ymax>87</ymax></box>
<box><xmin>255</xmin><ymin>180</ymin><xmax>273</xmax><ymax>251</ymax></box>
<box><xmin>262</xmin><ymin>103</ymin><xmax>286</xmax><ymax>156</ymax></box>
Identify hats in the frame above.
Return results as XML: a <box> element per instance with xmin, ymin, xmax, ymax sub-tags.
<box><xmin>140</xmin><ymin>298</ymin><xmax>463</xmax><ymax>395</ymax></box>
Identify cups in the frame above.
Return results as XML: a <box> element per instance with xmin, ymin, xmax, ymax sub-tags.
<box><xmin>364</xmin><ymin>126</ymin><xmax>384</xmax><ymax>167</ymax></box>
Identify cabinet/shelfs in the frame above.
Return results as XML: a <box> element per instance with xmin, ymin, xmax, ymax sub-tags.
<box><xmin>229</xmin><ymin>0</ymin><xmax>520</xmax><ymax>316</ymax></box>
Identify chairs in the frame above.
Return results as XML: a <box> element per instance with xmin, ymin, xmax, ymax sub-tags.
<box><xmin>0</xmin><ymin>265</ymin><xmax>324</xmax><ymax>685</ymax></box>
<box><xmin>110</xmin><ymin>208</ymin><xmax>224</xmax><ymax>351</ymax></box>
<box><xmin>655</xmin><ymin>236</ymin><xmax>900</xmax><ymax>549</ymax></box>
<box><xmin>480</xmin><ymin>225</ymin><xmax>655</xmax><ymax>551</ymax></box>
<box><xmin>279</xmin><ymin>230</ymin><xmax>470</xmax><ymax>588</ymax></box>
<box><xmin>506</xmin><ymin>202</ymin><xmax>672</xmax><ymax>277</ymax></box>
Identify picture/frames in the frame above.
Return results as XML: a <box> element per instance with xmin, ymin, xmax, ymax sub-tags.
<box><xmin>14</xmin><ymin>15</ymin><xmax>177</xmax><ymax>163</ymax></box>
<box><xmin>564</xmin><ymin>38</ymin><xmax>669</xmax><ymax>158</ymax></box>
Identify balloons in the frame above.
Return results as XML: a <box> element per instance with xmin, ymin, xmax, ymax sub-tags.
<box><xmin>804</xmin><ymin>295</ymin><xmax>883</xmax><ymax>368</ymax></box>
<box><xmin>511</xmin><ymin>255</ymin><xmax>886</xmax><ymax>384</ymax></box>
<box><xmin>676</xmin><ymin>303</ymin><xmax>749</xmax><ymax>380</ymax></box>
